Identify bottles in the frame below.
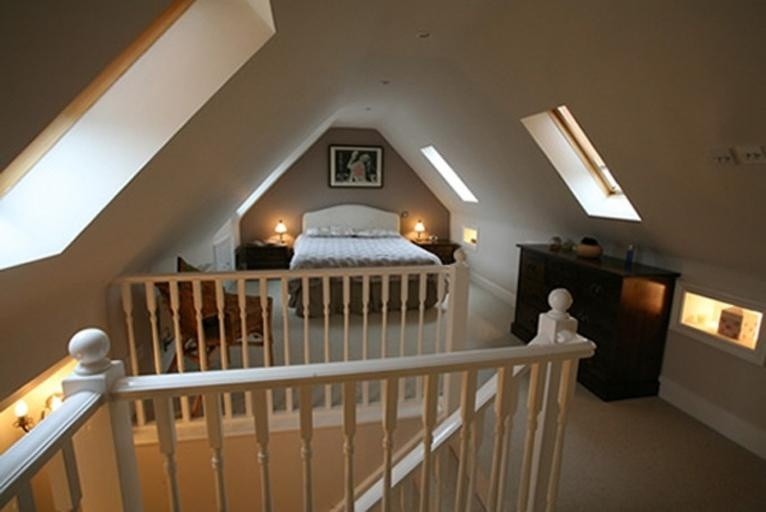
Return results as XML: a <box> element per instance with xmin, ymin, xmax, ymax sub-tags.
<box><xmin>625</xmin><ymin>244</ymin><xmax>634</xmax><ymax>271</ymax></box>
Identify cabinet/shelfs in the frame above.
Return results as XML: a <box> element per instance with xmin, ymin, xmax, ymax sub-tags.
<box><xmin>511</xmin><ymin>241</ymin><xmax>683</xmax><ymax>403</ymax></box>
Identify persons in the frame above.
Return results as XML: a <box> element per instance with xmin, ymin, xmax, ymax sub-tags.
<box><xmin>346</xmin><ymin>150</ymin><xmax>372</xmax><ymax>183</ymax></box>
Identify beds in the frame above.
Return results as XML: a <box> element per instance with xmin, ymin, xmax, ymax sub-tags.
<box><xmin>295</xmin><ymin>202</ymin><xmax>445</xmax><ymax>319</ymax></box>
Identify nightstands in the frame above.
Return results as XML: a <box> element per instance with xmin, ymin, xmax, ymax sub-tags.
<box><xmin>408</xmin><ymin>236</ymin><xmax>460</xmax><ymax>265</ymax></box>
<box><xmin>241</xmin><ymin>243</ymin><xmax>288</xmax><ymax>271</ymax></box>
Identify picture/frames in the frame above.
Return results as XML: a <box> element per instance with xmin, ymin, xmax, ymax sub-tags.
<box><xmin>325</xmin><ymin>142</ymin><xmax>385</xmax><ymax>190</ymax></box>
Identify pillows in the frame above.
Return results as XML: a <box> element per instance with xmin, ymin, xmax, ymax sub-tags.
<box><xmin>305</xmin><ymin>217</ymin><xmax>402</xmax><ymax>239</ymax></box>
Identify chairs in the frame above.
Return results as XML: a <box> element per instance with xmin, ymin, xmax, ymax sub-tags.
<box><xmin>154</xmin><ymin>279</ymin><xmax>276</xmax><ymax>418</ymax></box>
